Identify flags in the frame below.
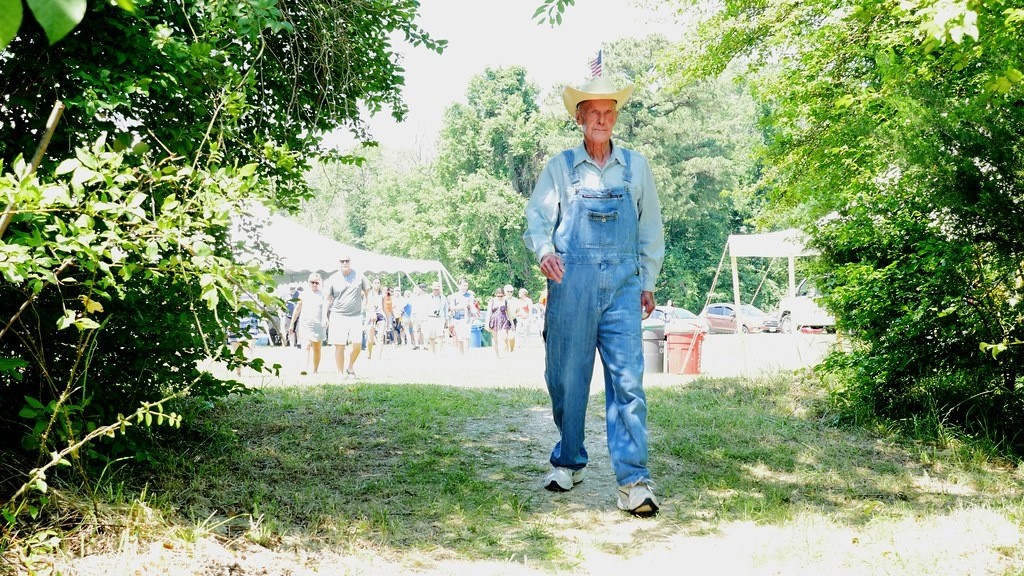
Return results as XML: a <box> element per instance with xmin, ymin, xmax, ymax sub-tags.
<box><xmin>588</xmin><ymin>50</ymin><xmax>602</xmax><ymax>78</ymax></box>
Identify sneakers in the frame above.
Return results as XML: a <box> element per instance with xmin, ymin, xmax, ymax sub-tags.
<box><xmin>616</xmin><ymin>476</ymin><xmax>660</xmax><ymax>516</ymax></box>
<box><xmin>543</xmin><ymin>465</ymin><xmax>587</xmax><ymax>491</ymax></box>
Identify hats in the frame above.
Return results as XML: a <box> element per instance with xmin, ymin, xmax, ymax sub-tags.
<box><xmin>561</xmin><ymin>77</ymin><xmax>634</xmax><ymax>118</ymax></box>
<box><xmin>432</xmin><ymin>282</ymin><xmax>441</xmax><ymax>290</ymax></box>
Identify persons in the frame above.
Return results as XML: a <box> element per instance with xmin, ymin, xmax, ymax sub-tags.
<box><xmin>362</xmin><ymin>276</ymin><xmax>481</xmax><ymax>360</ymax></box>
<box><xmin>484</xmin><ymin>284</ymin><xmax>533</xmax><ymax>359</ymax></box>
<box><xmin>284</xmin><ymin>272</ymin><xmax>327</xmax><ymax>375</ymax></box>
<box><xmin>522</xmin><ymin>77</ymin><xmax>665</xmax><ymax>514</ymax></box>
<box><xmin>326</xmin><ymin>253</ymin><xmax>371</xmax><ymax>382</ymax></box>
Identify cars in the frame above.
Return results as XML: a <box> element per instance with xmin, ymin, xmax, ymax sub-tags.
<box><xmin>695</xmin><ymin>302</ymin><xmax>778</xmax><ymax>335</ymax></box>
<box><xmin>650</xmin><ymin>305</ymin><xmax>707</xmax><ymax>337</ymax></box>
<box><xmin>778</xmin><ymin>280</ymin><xmax>851</xmax><ymax>334</ymax></box>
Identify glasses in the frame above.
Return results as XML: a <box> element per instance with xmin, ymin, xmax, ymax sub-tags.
<box><xmin>340</xmin><ymin>260</ymin><xmax>350</xmax><ymax>263</ymax></box>
<box><xmin>310</xmin><ymin>281</ymin><xmax>319</xmax><ymax>285</ymax></box>
<box><xmin>507</xmin><ymin>291</ymin><xmax>513</xmax><ymax>293</ymax></box>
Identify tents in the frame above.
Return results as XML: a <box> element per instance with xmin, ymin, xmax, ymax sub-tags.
<box><xmin>227</xmin><ymin>212</ymin><xmax>449</xmax><ymax>357</ymax></box>
<box><xmin>728</xmin><ymin>165</ymin><xmax>957</xmax><ymax>344</ymax></box>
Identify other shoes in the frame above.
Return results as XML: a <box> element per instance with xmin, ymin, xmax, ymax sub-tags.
<box><xmin>345</xmin><ymin>369</ymin><xmax>356</xmax><ymax>378</ymax></box>
